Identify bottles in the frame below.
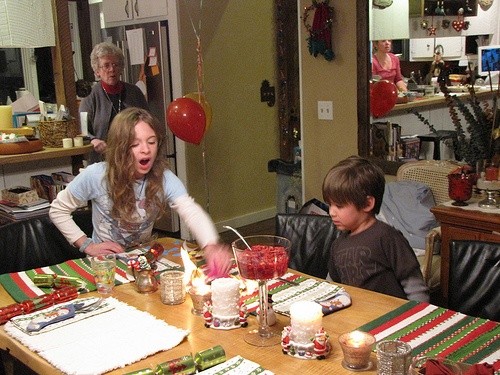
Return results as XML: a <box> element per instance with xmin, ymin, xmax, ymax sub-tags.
<box><xmin>435</xmin><ymin>1</ymin><xmax>444</xmax><ymax>15</ymax></box>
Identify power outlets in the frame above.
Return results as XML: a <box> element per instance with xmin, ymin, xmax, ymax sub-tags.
<box><xmin>317</xmin><ymin>101</ymin><xmax>333</xmax><ymax>120</ymax></box>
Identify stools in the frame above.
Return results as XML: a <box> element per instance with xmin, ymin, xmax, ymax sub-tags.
<box><xmin>418</xmin><ymin>130</ymin><xmax>462</xmax><ymax>160</ymax></box>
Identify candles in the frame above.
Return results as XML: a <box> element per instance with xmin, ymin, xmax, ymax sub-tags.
<box><xmin>348</xmin><ymin>331</ymin><xmax>366</xmax><ymax>347</ymax></box>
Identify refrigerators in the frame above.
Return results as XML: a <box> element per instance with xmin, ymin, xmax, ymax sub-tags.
<box><xmin>99</xmin><ymin>22</ymin><xmax>179</xmax><ymax>233</ymax></box>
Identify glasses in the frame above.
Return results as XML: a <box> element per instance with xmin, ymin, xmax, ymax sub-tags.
<box><xmin>98</xmin><ymin>62</ymin><xmax>123</xmax><ymax>71</ymax></box>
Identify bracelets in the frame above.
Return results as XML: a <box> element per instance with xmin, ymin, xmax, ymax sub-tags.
<box><xmin>79</xmin><ymin>238</ymin><xmax>92</xmax><ymax>252</ymax></box>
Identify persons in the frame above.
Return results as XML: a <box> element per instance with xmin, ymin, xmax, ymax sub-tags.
<box><xmin>320</xmin><ymin>155</ymin><xmax>430</xmax><ymax>306</ymax></box>
<box><xmin>46</xmin><ymin>107</ymin><xmax>234</xmax><ymax>276</ymax></box>
<box><xmin>371</xmin><ymin>39</ymin><xmax>409</xmax><ymax>93</ymax></box>
<box><xmin>78</xmin><ymin>38</ymin><xmax>149</xmax><ymax>168</ymax></box>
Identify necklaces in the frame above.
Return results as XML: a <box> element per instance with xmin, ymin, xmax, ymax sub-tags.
<box><xmin>100</xmin><ymin>83</ymin><xmax>122</xmax><ymax>116</ymax></box>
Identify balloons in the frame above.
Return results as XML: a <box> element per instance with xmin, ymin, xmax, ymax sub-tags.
<box><xmin>370</xmin><ymin>83</ymin><xmax>399</xmax><ymax>118</ymax></box>
<box><xmin>166</xmin><ymin>97</ymin><xmax>206</xmax><ymax>146</ymax></box>
<box><xmin>183</xmin><ymin>93</ymin><xmax>212</xmax><ymax>134</ymax></box>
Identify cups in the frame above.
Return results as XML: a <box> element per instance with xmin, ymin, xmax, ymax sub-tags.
<box><xmin>160</xmin><ymin>271</ymin><xmax>187</xmax><ymax>305</ymax></box>
<box><xmin>448</xmin><ymin>173</ymin><xmax>471</xmax><ymax>206</ymax></box>
<box><xmin>188</xmin><ymin>285</ymin><xmax>212</xmax><ymax>316</ymax></box>
<box><xmin>337</xmin><ymin>331</ymin><xmax>376</xmax><ymax>371</ymax></box>
<box><xmin>376</xmin><ymin>340</ymin><xmax>412</xmax><ymax>375</ymax></box>
<box><xmin>91</xmin><ymin>254</ymin><xmax>116</xmax><ymax>294</ymax></box>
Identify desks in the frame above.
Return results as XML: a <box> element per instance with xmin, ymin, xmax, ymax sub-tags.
<box><xmin>0</xmin><ymin>143</ymin><xmax>94</xmax><ymax>175</ymax></box>
<box><xmin>370</xmin><ymin>88</ymin><xmax>500</xmax><ymax>142</ymax></box>
<box><xmin>430</xmin><ymin>201</ymin><xmax>500</xmax><ymax>308</ymax></box>
<box><xmin>0</xmin><ymin>238</ymin><xmax>500</xmax><ymax>375</ymax></box>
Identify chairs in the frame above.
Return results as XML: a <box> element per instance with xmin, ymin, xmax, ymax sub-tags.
<box><xmin>0</xmin><ymin>211</ymin><xmax>93</xmax><ymax>276</ymax></box>
<box><xmin>274</xmin><ymin>160</ymin><xmax>500</xmax><ymax>323</ymax></box>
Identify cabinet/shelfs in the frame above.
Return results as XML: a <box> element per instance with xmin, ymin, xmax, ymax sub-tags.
<box><xmin>409</xmin><ymin>16</ymin><xmax>466</xmax><ymax>62</ymax></box>
<box><xmin>102</xmin><ymin>0</ymin><xmax>168</xmax><ymax>23</ymax></box>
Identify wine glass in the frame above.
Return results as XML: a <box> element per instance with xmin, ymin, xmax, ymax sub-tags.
<box><xmin>232</xmin><ymin>235</ymin><xmax>292</xmax><ymax>347</ymax></box>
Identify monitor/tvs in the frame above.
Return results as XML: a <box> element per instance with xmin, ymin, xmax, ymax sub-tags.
<box><xmin>478</xmin><ymin>45</ymin><xmax>500</xmax><ymax>77</ymax></box>
